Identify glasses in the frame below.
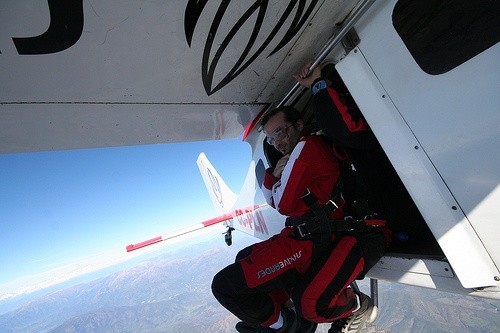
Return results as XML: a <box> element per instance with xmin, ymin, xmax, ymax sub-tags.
<box><xmin>267</xmin><ymin>123</ymin><xmax>295</xmax><ymax>145</ymax></box>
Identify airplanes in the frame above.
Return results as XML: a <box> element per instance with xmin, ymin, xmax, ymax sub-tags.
<box><xmin>0</xmin><ymin>0</ymin><xmax>500</xmax><ymax>333</ymax></box>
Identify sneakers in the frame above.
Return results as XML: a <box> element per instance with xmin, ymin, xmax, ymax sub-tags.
<box><xmin>235</xmin><ymin>303</ymin><xmax>297</xmax><ymax>333</ymax></box>
<box><xmin>328</xmin><ymin>291</ymin><xmax>378</xmax><ymax>333</ymax></box>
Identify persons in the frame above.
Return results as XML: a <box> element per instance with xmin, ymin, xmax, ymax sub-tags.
<box><xmin>291</xmin><ymin>60</ymin><xmax>393</xmax><ymax>333</ymax></box>
<box><xmin>211</xmin><ymin>106</ymin><xmax>347</xmax><ymax>333</ymax></box>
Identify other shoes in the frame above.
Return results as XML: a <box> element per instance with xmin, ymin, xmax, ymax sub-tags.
<box><xmin>295</xmin><ymin>306</ymin><xmax>317</xmax><ymax>333</ymax></box>
